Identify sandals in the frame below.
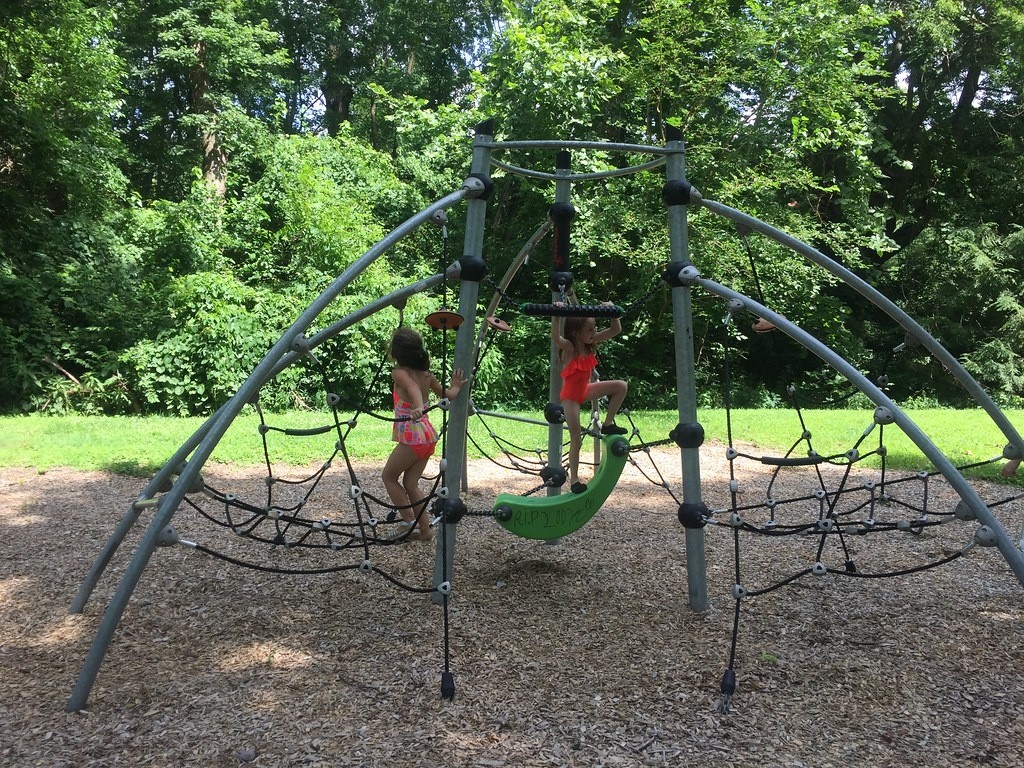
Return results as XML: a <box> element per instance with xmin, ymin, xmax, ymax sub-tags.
<box><xmin>384</xmin><ymin>520</ymin><xmax>437</xmax><ymax>544</ymax></box>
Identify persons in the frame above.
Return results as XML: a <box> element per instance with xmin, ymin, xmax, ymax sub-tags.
<box><xmin>552</xmin><ymin>302</ymin><xmax>627</xmax><ymax>494</ymax></box>
<box><xmin>382</xmin><ymin>328</ymin><xmax>468</xmax><ymax>542</ymax></box>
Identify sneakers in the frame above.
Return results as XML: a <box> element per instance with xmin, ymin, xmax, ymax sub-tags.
<box><xmin>601</xmin><ymin>418</ymin><xmax>628</xmax><ymax>434</ymax></box>
<box><xmin>571</xmin><ymin>480</ymin><xmax>587</xmax><ymax>494</ymax></box>
